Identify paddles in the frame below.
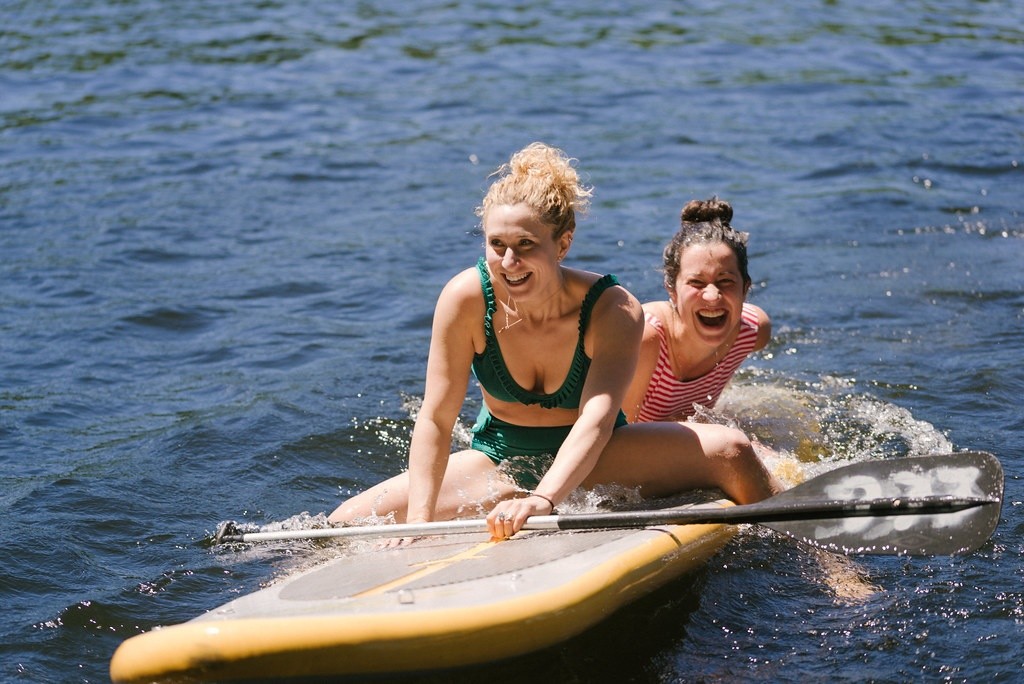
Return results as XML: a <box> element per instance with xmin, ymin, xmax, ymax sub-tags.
<box><xmin>214</xmin><ymin>448</ymin><xmax>1007</xmax><ymax>557</ymax></box>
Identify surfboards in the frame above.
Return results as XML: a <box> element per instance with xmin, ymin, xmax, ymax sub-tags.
<box><xmin>107</xmin><ymin>438</ymin><xmax>808</xmax><ymax>684</ymax></box>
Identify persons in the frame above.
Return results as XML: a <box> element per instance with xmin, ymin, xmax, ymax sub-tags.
<box><xmin>621</xmin><ymin>196</ymin><xmax>771</xmax><ymax>424</ymax></box>
<box><xmin>328</xmin><ymin>142</ymin><xmax>786</xmax><ymax>550</ymax></box>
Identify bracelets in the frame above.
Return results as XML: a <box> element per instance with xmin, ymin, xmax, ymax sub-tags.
<box><xmin>528</xmin><ymin>494</ymin><xmax>554</xmax><ymax>511</ymax></box>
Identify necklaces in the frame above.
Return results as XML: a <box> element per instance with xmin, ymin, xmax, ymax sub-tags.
<box><xmin>499</xmin><ymin>272</ymin><xmax>566</xmax><ymax>333</ymax></box>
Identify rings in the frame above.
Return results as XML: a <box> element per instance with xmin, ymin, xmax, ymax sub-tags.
<box><xmin>497</xmin><ymin>512</ymin><xmax>506</xmax><ymax>522</ymax></box>
<box><xmin>506</xmin><ymin>512</ymin><xmax>515</xmax><ymax>523</ymax></box>
